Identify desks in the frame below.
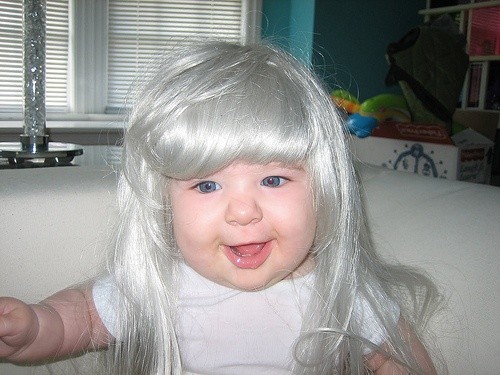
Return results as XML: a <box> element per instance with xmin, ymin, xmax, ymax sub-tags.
<box><xmin>0</xmin><ymin>142</ymin><xmax>126</xmax><ymax>172</ymax></box>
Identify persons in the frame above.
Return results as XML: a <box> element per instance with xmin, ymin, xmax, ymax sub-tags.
<box><xmin>0</xmin><ymin>39</ymin><xmax>438</xmax><ymax>375</ymax></box>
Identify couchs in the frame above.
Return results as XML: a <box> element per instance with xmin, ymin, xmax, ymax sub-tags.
<box><xmin>0</xmin><ymin>163</ymin><xmax>500</xmax><ymax>375</ymax></box>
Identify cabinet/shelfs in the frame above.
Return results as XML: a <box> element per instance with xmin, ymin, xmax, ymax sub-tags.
<box><xmin>418</xmin><ymin>0</ymin><xmax>500</xmax><ymax>111</ymax></box>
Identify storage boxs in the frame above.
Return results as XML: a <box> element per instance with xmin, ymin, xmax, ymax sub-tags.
<box><xmin>350</xmin><ymin>121</ymin><xmax>494</xmax><ymax>184</ymax></box>
